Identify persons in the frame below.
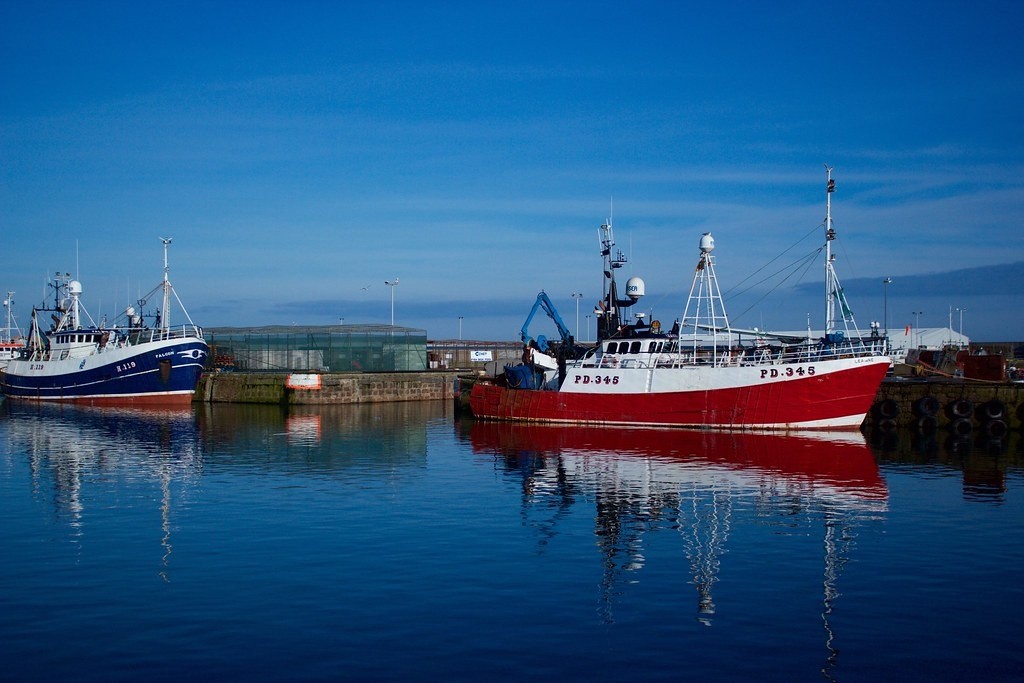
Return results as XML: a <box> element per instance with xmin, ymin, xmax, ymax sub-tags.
<box><xmin>1010</xmin><ymin>362</ymin><xmax>1016</xmax><ymax>379</ymax></box>
<box><xmin>806</xmin><ymin>348</ymin><xmax>815</xmax><ymax>362</ymax></box>
<box><xmin>763</xmin><ymin>346</ymin><xmax>770</xmax><ymax>360</ymax></box>
<box><xmin>155</xmin><ymin>311</ymin><xmax>161</xmax><ymax>328</ymax></box>
<box><xmin>101</xmin><ymin>333</ymin><xmax>109</xmax><ymax>347</ymax></box>
<box><xmin>353</xmin><ymin>354</ymin><xmax>361</xmax><ymax>370</ymax></box>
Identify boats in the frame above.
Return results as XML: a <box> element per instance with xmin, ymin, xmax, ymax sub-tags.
<box><xmin>470</xmin><ymin>416</ymin><xmax>890</xmax><ymax>669</ymax></box>
<box><xmin>1</xmin><ymin>392</ymin><xmax>198</xmax><ymax>584</ymax></box>
<box><xmin>469</xmin><ymin>165</ymin><xmax>892</xmax><ymax>434</ymax></box>
<box><xmin>453</xmin><ymin>374</ymin><xmax>491</xmax><ymax>413</ymax></box>
<box><xmin>0</xmin><ymin>237</ymin><xmax>209</xmax><ymax>407</ymax></box>
<box><xmin>887</xmin><ymin>349</ymin><xmax>906</xmax><ymax>367</ymax></box>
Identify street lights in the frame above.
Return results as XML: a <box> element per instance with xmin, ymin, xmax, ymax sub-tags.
<box><xmin>339</xmin><ymin>318</ymin><xmax>344</xmax><ymax>334</ymax></box>
<box><xmin>459</xmin><ymin>316</ymin><xmax>463</xmax><ymax>340</ymax></box>
<box><xmin>883</xmin><ymin>278</ymin><xmax>892</xmax><ymax>333</ymax></box>
<box><xmin>384</xmin><ymin>278</ymin><xmax>399</xmax><ymax>345</ymax></box>
<box><xmin>956</xmin><ymin>308</ymin><xmax>967</xmax><ymax>351</ymax></box>
<box><xmin>911</xmin><ymin>312</ymin><xmax>923</xmax><ymax>348</ymax></box>
